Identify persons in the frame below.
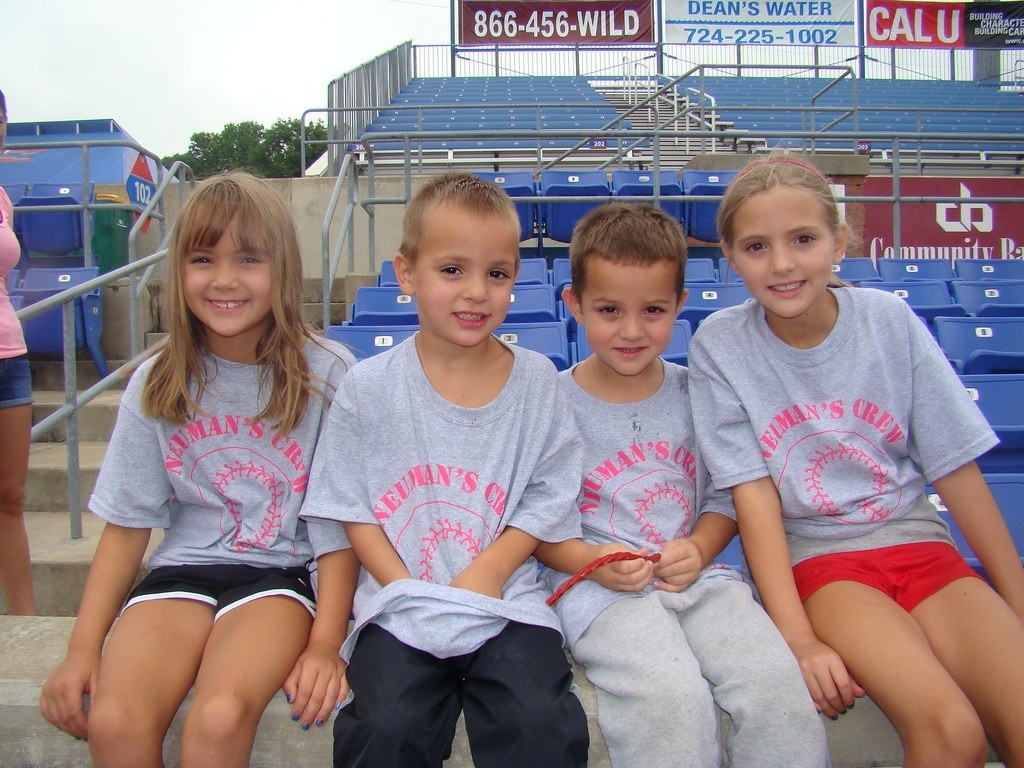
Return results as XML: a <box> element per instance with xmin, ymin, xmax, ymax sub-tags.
<box><xmin>687</xmin><ymin>154</ymin><xmax>1024</xmax><ymax>767</ymax></box>
<box><xmin>39</xmin><ymin>174</ymin><xmax>357</xmax><ymax>768</ymax></box>
<box><xmin>298</xmin><ymin>171</ymin><xmax>586</xmax><ymax>768</ymax></box>
<box><xmin>0</xmin><ymin>90</ymin><xmax>37</xmax><ymax>618</ymax></box>
<box><xmin>532</xmin><ymin>202</ymin><xmax>831</xmax><ymax>768</ymax></box>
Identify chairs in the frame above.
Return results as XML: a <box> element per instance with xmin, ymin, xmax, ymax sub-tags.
<box><xmin>0</xmin><ymin>72</ymin><xmax>1024</xmax><ymax>570</ymax></box>
